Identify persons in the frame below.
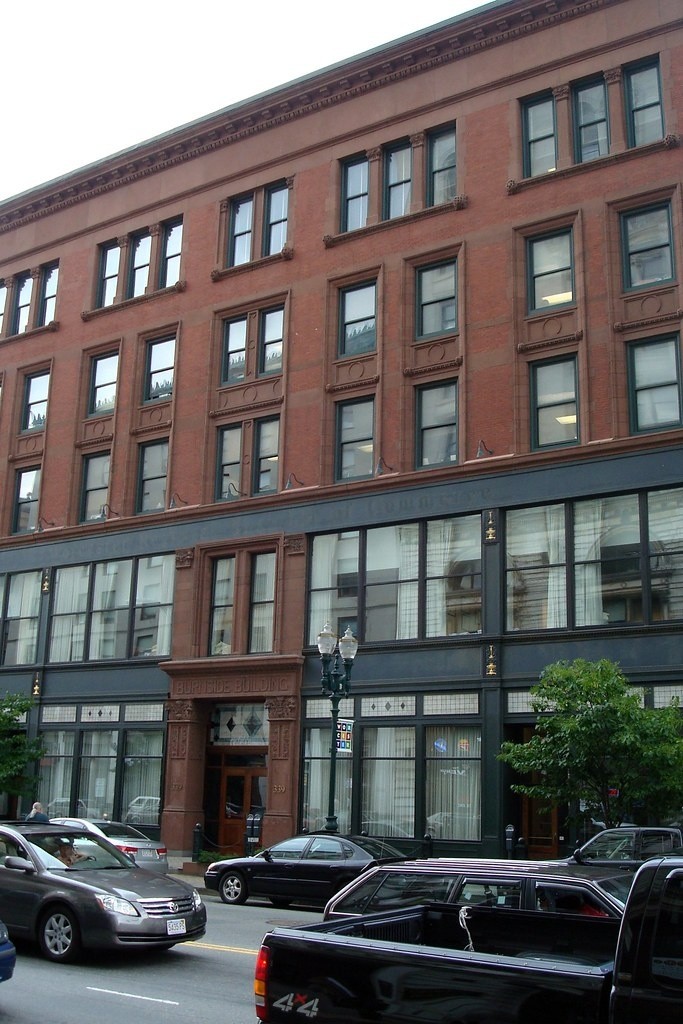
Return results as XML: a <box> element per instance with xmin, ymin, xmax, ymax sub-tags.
<box><xmin>58</xmin><ymin>842</ymin><xmax>88</xmax><ymax>866</ymax></box>
<box><xmin>25</xmin><ymin>802</ymin><xmax>50</xmax><ymax>823</ymax></box>
<box><xmin>580</xmin><ymin>890</ymin><xmax>609</xmax><ymax>916</ymax></box>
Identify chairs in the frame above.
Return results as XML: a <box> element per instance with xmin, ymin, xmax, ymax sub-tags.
<box><xmin>0</xmin><ymin>843</ymin><xmax>27</xmax><ymax>865</ymax></box>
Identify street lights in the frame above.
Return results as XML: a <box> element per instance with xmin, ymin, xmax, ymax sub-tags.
<box><xmin>314</xmin><ymin>620</ymin><xmax>358</xmax><ymax>833</ymax></box>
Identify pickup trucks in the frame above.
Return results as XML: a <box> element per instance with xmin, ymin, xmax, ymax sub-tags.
<box><xmin>254</xmin><ymin>853</ymin><xmax>683</xmax><ymax>1024</ymax></box>
<box><xmin>556</xmin><ymin>826</ymin><xmax>683</xmax><ymax>872</ymax></box>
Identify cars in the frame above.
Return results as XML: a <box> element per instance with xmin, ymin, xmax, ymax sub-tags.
<box><xmin>48</xmin><ymin>816</ymin><xmax>168</xmax><ymax>874</ymax></box>
<box><xmin>345</xmin><ymin>820</ymin><xmax>415</xmax><ymax>838</ymax></box>
<box><xmin>204</xmin><ymin>832</ymin><xmax>406</xmax><ymax>906</ymax></box>
<box><xmin>0</xmin><ymin>921</ymin><xmax>15</xmax><ymax>984</ymax></box>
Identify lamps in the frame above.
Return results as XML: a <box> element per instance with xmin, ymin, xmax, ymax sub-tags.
<box><xmin>376</xmin><ymin>457</ymin><xmax>393</xmax><ymax>474</ymax></box>
<box><xmin>476</xmin><ymin>439</ymin><xmax>492</xmax><ymax>458</ymax></box>
<box><xmin>286</xmin><ymin>472</ymin><xmax>303</xmax><ymax>490</ymax></box>
<box><xmin>37</xmin><ymin>515</ymin><xmax>54</xmax><ymax>534</ymax></box>
<box><xmin>99</xmin><ymin>504</ymin><xmax>119</xmax><ymax>520</ymax></box>
<box><xmin>226</xmin><ymin>482</ymin><xmax>248</xmax><ymax>499</ymax></box>
<box><xmin>169</xmin><ymin>493</ymin><xmax>187</xmax><ymax>509</ymax></box>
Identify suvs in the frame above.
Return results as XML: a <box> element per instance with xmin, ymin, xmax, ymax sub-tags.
<box><xmin>0</xmin><ymin>819</ymin><xmax>207</xmax><ymax>962</ymax></box>
<box><xmin>320</xmin><ymin>858</ymin><xmax>637</xmax><ymax>922</ymax></box>
<box><xmin>423</xmin><ymin>810</ymin><xmax>480</xmax><ymax>840</ymax></box>
<box><xmin>45</xmin><ymin>798</ymin><xmax>101</xmax><ymax>819</ymax></box>
<box><xmin>125</xmin><ymin>795</ymin><xmax>160</xmax><ymax>825</ymax></box>
